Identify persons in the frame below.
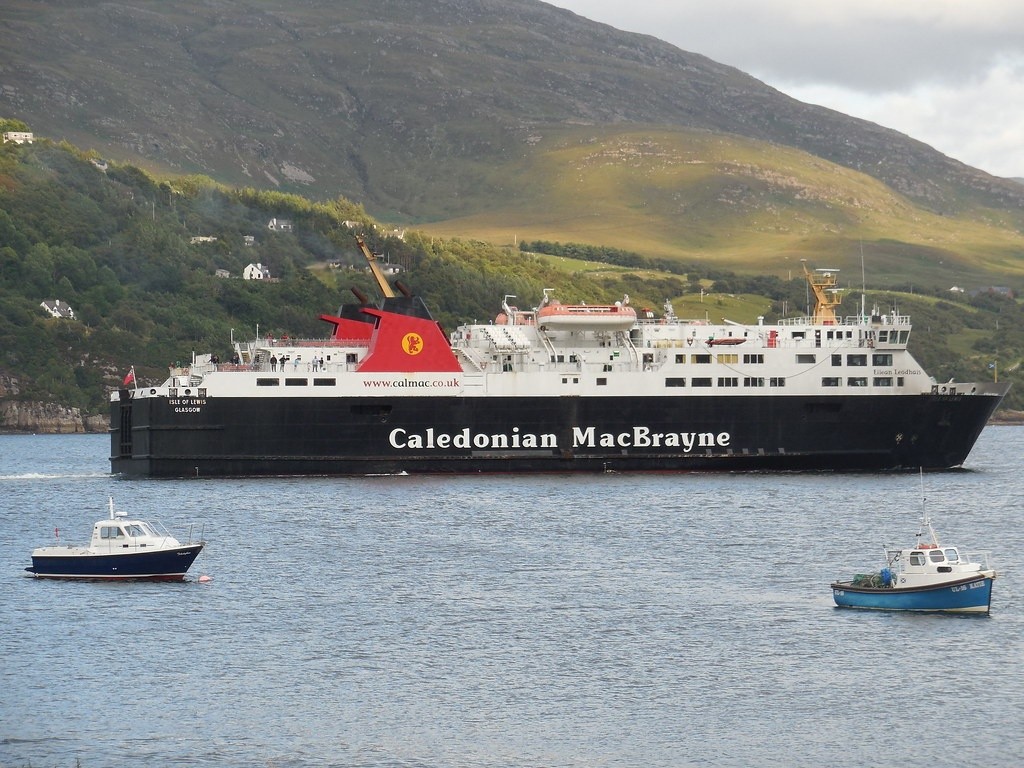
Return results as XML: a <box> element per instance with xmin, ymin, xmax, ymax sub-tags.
<box><xmin>209</xmin><ymin>355</ymin><xmax>219</xmax><ymax>364</ymax></box>
<box><xmin>267</xmin><ymin>332</ymin><xmax>273</xmax><ymax>347</ymax></box>
<box><xmin>281</xmin><ymin>332</ymin><xmax>288</xmax><ymax>346</ymax></box>
<box><xmin>270</xmin><ymin>354</ymin><xmax>324</xmax><ymax>373</ymax></box>
<box><xmin>231</xmin><ymin>354</ymin><xmax>239</xmax><ymax>364</ymax></box>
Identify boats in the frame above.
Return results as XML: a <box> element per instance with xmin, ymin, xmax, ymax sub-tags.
<box><xmin>105</xmin><ymin>221</ymin><xmax>1004</xmax><ymax>475</ymax></box>
<box><xmin>23</xmin><ymin>494</ymin><xmax>206</xmax><ymax>583</ymax></box>
<box><xmin>829</xmin><ymin>466</ymin><xmax>998</xmax><ymax>616</ymax></box>
